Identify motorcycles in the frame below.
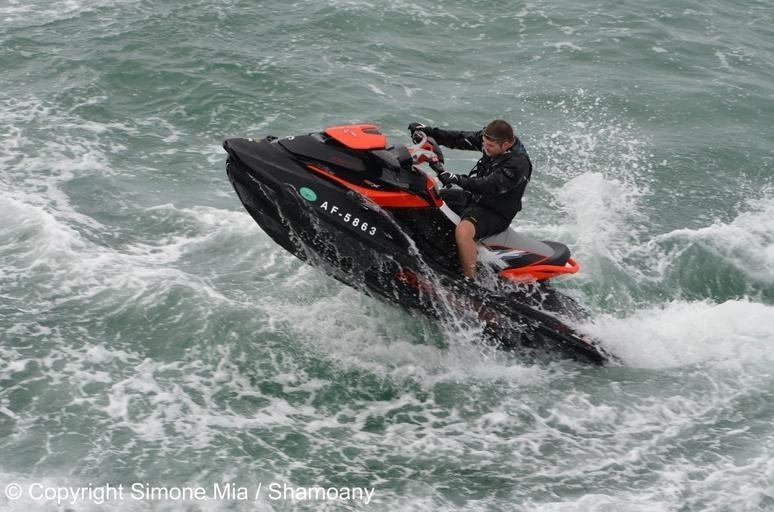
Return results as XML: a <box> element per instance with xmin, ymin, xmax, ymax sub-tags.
<box><xmin>217</xmin><ymin>120</ymin><xmax>626</xmax><ymax>370</ymax></box>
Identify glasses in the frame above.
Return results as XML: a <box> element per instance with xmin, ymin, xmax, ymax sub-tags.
<box><xmin>481</xmin><ymin>127</ymin><xmax>504</xmax><ymax>142</ymax></box>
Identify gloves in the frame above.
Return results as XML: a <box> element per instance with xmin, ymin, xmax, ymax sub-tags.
<box><xmin>408</xmin><ymin>121</ymin><xmax>425</xmax><ymax>133</ymax></box>
<box><xmin>437</xmin><ymin>169</ymin><xmax>459</xmax><ymax>186</ymax></box>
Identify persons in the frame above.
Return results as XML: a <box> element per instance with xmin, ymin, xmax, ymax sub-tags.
<box><xmin>406</xmin><ymin>118</ymin><xmax>534</xmax><ymax>281</ymax></box>
